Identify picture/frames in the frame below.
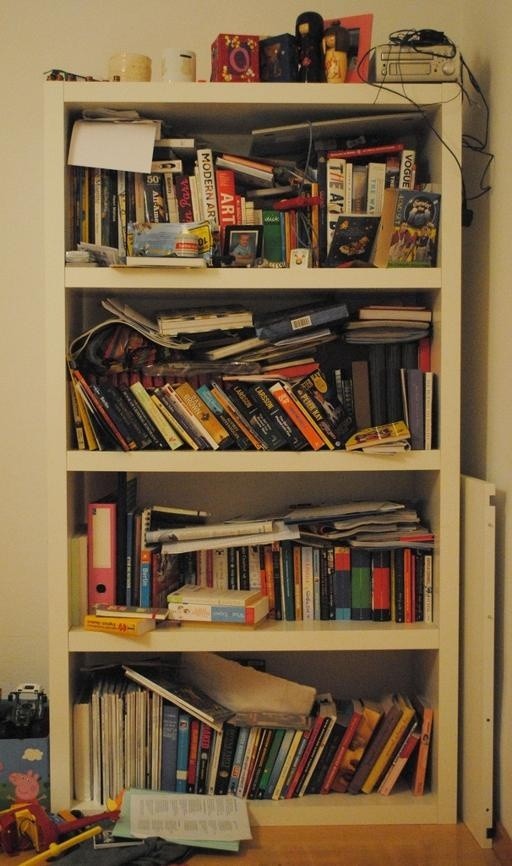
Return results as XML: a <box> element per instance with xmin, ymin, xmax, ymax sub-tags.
<box><xmin>224</xmin><ymin>224</ymin><xmax>261</xmax><ymax>263</ymax></box>
<box><xmin>316</xmin><ymin>13</ymin><xmax>373</xmax><ymax>81</ymax></box>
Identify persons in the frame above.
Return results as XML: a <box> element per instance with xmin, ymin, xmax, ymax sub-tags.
<box><xmin>324</xmin><ymin>20</ymin><xmax>350</xmax><ymax>83</ymax></box>
<box><xmin>295</xmin><ymin>12</ymin><xmax>324</xmax><ymax>82</ymax></box>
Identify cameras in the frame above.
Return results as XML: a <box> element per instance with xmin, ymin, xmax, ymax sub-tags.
<box><xmin>0</xmin><ymin>683</ymin><xmax>49</xmax><ymax>739</ymax></box>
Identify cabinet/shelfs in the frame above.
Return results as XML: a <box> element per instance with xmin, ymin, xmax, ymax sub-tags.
<box><xmin>45</xmin><ymin>80</ymin><xmax>462</xmax><ymax>824</ymax></box>
